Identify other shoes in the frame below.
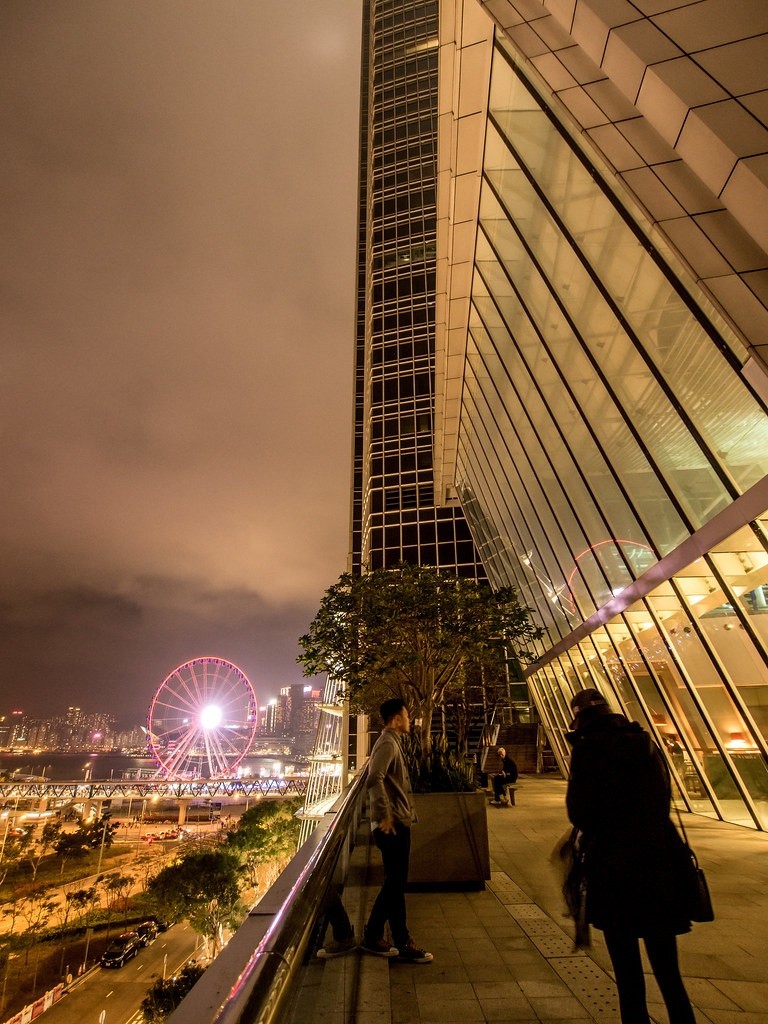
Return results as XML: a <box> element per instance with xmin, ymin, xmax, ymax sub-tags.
<box><xmin>504</xmin><ymin>797</ymin><xmax>510</xmax><ymax>805</ymax></box>
<box><xmin>491</xmin><ymin>800</ymin><xmax>502</xmax><ymax>805</ymax></box>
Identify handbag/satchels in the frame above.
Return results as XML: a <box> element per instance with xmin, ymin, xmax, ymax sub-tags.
<box><xmin>560</xmin><ymin>825</ymin><xmax>593</xmax><ymax>953</ymax></box>
<box><xmin>685</xmin><ymin>846</ymin><xmax>715</xmax><ymax>923</ymax></box>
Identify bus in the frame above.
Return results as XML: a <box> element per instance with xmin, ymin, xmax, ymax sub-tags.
<box><xmin>184</xmin><ymin>799</ymin><xmax>222</xmax><ymax>821</ymax></box>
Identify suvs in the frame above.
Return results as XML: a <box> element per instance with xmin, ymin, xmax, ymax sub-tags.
<box><xmin>98</xmin><ymin>931</ymin><xmax>140</xmax><ymax>968</ymax></box>
<box><xmin>133</xmin><ymin>921</ymin><xmax>159</xmax><ymax>948</ymax></box>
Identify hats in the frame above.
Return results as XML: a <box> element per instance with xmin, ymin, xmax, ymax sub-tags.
<box><xmin>570</xmin><ymin>688</ymin><xmax>609</xmax><ymax>719</ymax></box>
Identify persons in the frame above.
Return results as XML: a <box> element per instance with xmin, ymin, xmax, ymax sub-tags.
<box><xmin>360</xmin><ymin>698</ymin><xmax>433</xmax><ymax>964</ymax></box>
<box><xmin>490</xmin><ymin>748</ymin><xmax>518</xmax><ymax>807</ymax></box>
<box><xmin>315</xmin><ymin>897</ymin><xmax>357</xmax><ymax>960</ymax></box>
<box><xmin>564</xmin><ymin>688</ymin><xmax>698</xmax><ymax>1024</ymax></box>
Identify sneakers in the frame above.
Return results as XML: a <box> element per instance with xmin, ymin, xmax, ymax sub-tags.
<box><xmin>315</xmin><ymin>925</ymin><xmax>357</xmax><ymax>959</ymax></box>
<box><xmin>360</xmin><ymin>926</ymin><xmax>399</xmax><ymax>957</ymax></box>
<box><xmin>394</xmin><ymin>936</ymin><xmax>434</xmax><ymax>963</ymax></box>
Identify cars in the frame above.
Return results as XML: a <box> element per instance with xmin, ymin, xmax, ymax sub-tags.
<box><xmin>141</xmin><ymin>827</ymin><xmax>190</xmax><ymax>842</ymax></box>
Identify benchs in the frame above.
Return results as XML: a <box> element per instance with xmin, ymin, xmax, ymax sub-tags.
<box><xmin>488</xmin><ymin>776</ymin><xmax>523</xmax><ymax>809</ymax></box>
